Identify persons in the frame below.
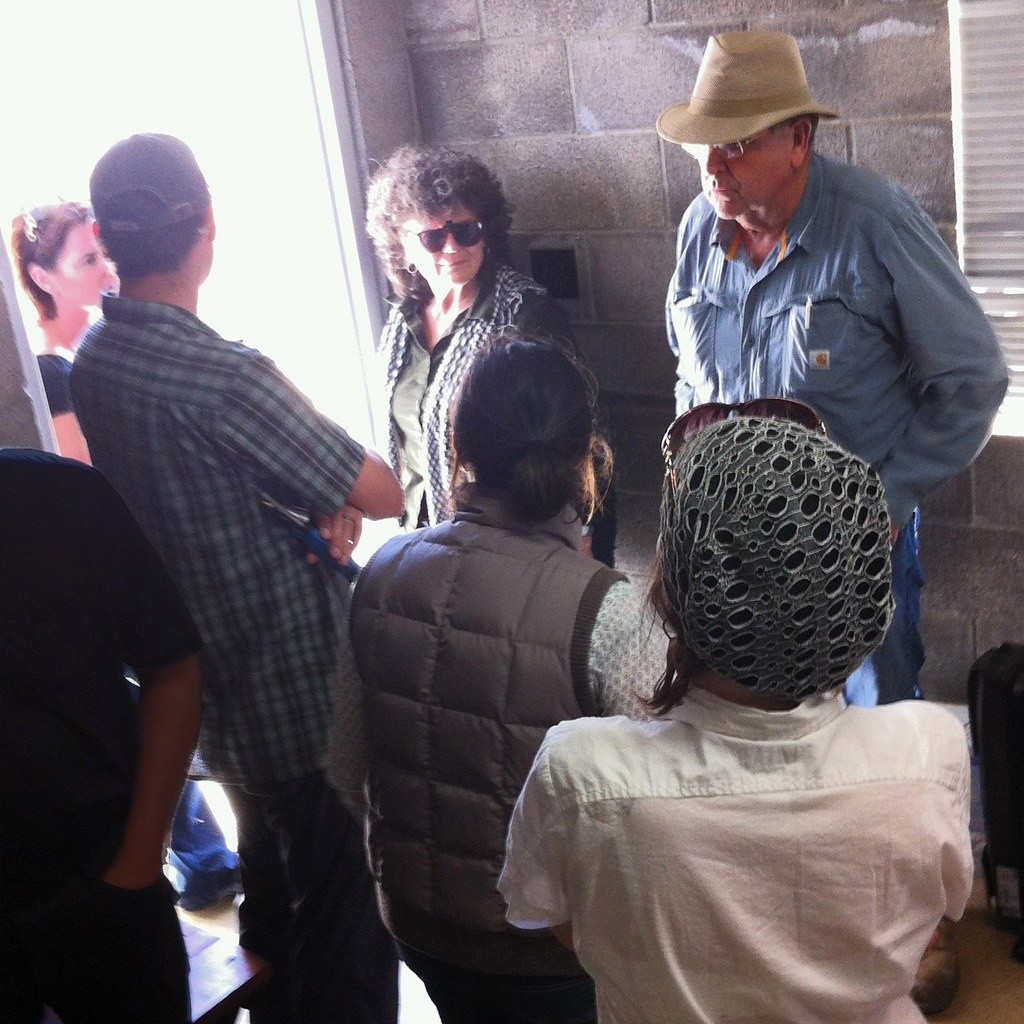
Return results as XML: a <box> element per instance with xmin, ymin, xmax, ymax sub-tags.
<box><xmin>12</xmin><ymin>202</ymin><xmax>116</xmax><ymax>469</ymax></box>
<box><xmin>664</xmin><ymin>34</ymin><xmax>1009</xmax><ymax>707</ymax></box>
<box><xmin>0</xmin><ymin>449</ymin><xmax>211</xmax><ymax>1024</ymax></box>
<box><xmin>323</xmin><ymin>346</ymin><xmax>680</xmax><ymax>1024</ymax></box>
<box><xmin>66</xmin><ymin>132</ymin><xmax>406</xmax><ymax>1024</ymax></box>
<box><xmin>363</xmin><ymin>147</ymin><xmax>618</xmax><ymax>566</ymax></box>
<box><xmin>496</xmin><ymin>400</ymin><xmax>981</xmax><ymax>1024</ymax></box>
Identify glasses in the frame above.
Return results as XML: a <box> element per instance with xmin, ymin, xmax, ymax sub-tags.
<box><xmin>403</xmin><ymin>218</ymin><xmax>482</xmax><ymax>253</ymax></box>
<box><xmin>661</xmin><ymin>397</ymin><xmax>826</xmax><ymax>524</ymax></box>
<box><xmin>683</xmin><ymin>130</ymin><xmax>769</xmax><ymax>158</ymax></box>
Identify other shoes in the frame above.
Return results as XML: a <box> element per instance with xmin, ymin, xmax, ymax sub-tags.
<box><xmin>182</xmin><ymin>871</ymin><xmax>243</xmax><ymax>910</ymax></box>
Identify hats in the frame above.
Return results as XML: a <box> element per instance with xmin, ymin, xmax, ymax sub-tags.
<box><xmin>660</xmin><ymin>416</ymin><xmax>894</xmax><ymax>699</ymax></box>
<box><xmin>657</xmin><ymin>29</ymin><xmax>840</xmax><ymax>147</ymax></box>
<box><xmin>89</xmin><ymin>132</ymin><xmax>211</xmax><ymax>233</ymax></box>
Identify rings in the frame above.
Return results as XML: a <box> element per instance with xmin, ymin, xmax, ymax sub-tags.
<box><xmin>346</xmin><ymin>539</ymin><xmax>354</xmax><ymax>544</ymax></box>
<box><xmin>343</xmin><ymin>514</ymin><xmax>354</xmax><ymax>522</ymax></box>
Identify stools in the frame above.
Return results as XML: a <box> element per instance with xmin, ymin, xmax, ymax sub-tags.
<box><xmin>177</xmin><ymin>916</ymin><xmax>262</xmax><ymax>1024</ymax></box>
